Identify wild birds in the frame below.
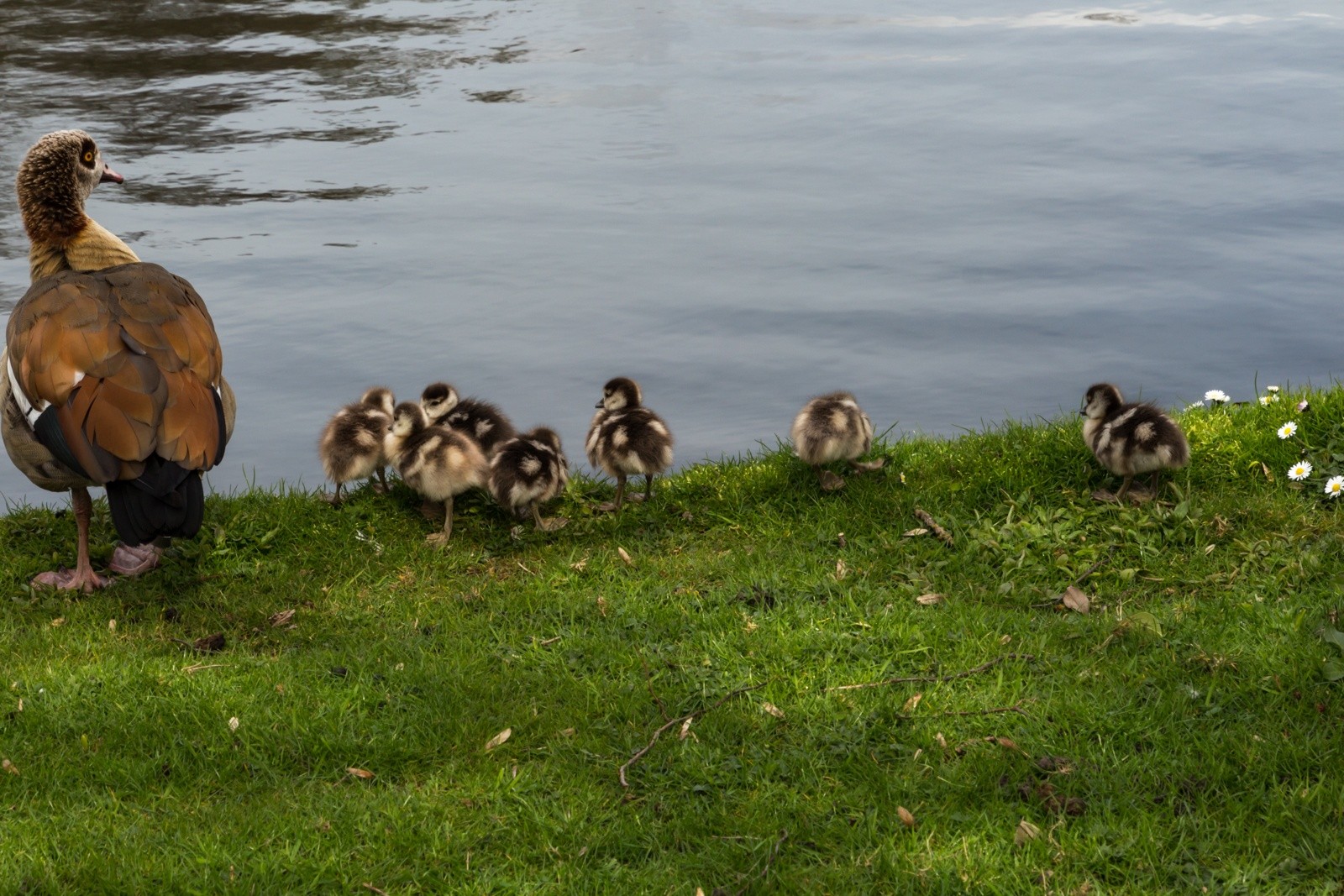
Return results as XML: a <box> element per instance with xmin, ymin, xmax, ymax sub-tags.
<box><xmin>584</xmin><ymin>376</ymin><xmax>677</xmax><ymax>512</ymax></box>
<box><xmin>790</xmin><ymin>391</ymin><xmax>885</xmax><ymax>491</ymax></box>
<box><xmin>0</xmin><ymin>128</ymin><xmax>237</xmax><ymax>594</ymax></box>
<box><xmin>1080</xmin><ymin>382</ymin><xmax>1190</xmax><ymax>504</ymax></box>
<box><xmin>314</xmin><ymin>381</ymin><xmax>573</xmax><ymax>546</ymax></box>
